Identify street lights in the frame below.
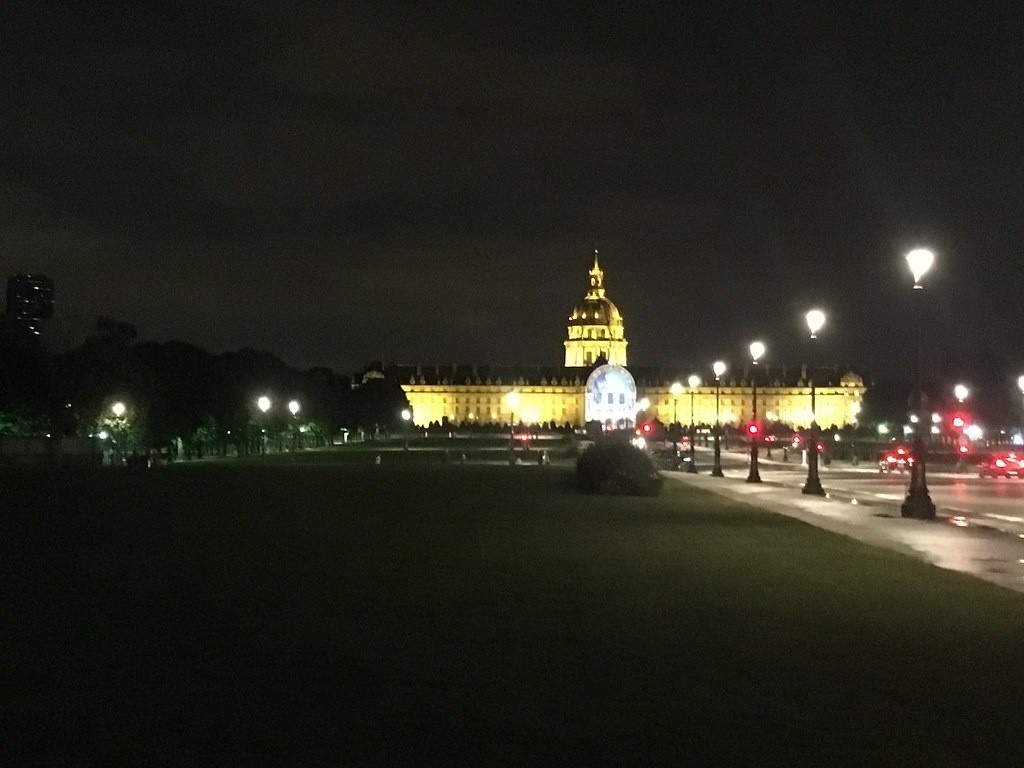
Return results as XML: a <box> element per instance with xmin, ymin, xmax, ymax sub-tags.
<box><xmin>747</xmin><ymin>342</ymin><xmax>765</xmax><ymax>483</ymax></box>
<box><xmin>711</xmin><ymin>360</ymin><xmax>727</xmax><ymax>477</ymax></box>
<box><xmin>900</xmin><ymin>246</ymin><xmax>936</xmax><ymax>520</ymax></box>
<box><xmin>802</xmin><ymin>308</ymin><xmax>825</xmax><ymax>496</ymax></box>
<box><xmin>668</xmin><ymin>382</ymin><xmax>683</xmax><ymax>470</ymax></box>
<box><xmin>686</xmin><ymin>375</ymin><xmax>700</xmax><ymax>474</ymax></box>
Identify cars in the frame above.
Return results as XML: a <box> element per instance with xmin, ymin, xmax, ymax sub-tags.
<box><xmin>879</xmin><ymin>449</ymin><xmax>912</xmax><ymax>473</ymax></box>
<box><xmin>978</xmin><ymin>451</ymin><xmax>1024</xmax><ymax>480</ymax></box>
